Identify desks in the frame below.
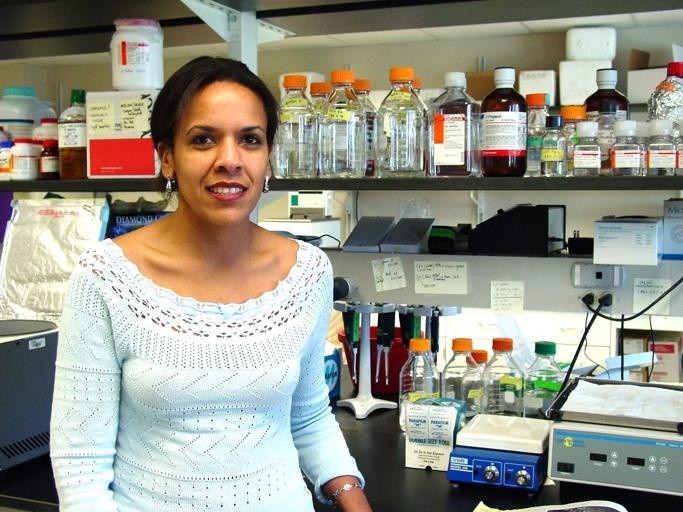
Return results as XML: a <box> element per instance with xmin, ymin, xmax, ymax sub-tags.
<box><xmin>0</xmin><ymin>404</ymin><xmax>683</xmax><ymax>512</ymax></box>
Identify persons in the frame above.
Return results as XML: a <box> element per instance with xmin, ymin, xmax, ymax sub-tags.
<box><xmin>47</xmin><ymin>55</ymin><xmax>374</xmax><ymax>512</ymax></box>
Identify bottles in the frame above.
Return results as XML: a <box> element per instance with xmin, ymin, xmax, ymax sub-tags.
<box><xmin>441</xmin><ymin>336</ymin><xmax>565</xmax><ymax>416</ymax></box>
<box><xmin>525</xmin><ymin>61</ymin><xmax>682</xmax><ymax>177</ymax></box>
<box><xmin>398</xmin><ymin>336</ymin><xmax>440</xmax><ymax>431</ymax></box>
<box><xmin>270</xmin><ymin>66</ymin><xmax>428</xmax><ymax>178</ymax></box>
<box><xmin>427</xmin><ymin>63</ymin><xmax>529</xmax><ymax>181</ymax></box>
<box><xmin>0</xmin><ymin>86</ymin><xmax>86</xmax><ymax>180</ymax></box>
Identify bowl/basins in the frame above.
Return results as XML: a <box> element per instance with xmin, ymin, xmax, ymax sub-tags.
<box><xmin>338</xmin><ymin>329</ymin><xmax>425</xmax><ymax>394</ymax></box>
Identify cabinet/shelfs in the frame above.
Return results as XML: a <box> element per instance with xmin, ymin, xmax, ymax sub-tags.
<box><xmin>0</xmin><ymin>0</ymin><xmax>683</xmax><ymax>261</ymax></box>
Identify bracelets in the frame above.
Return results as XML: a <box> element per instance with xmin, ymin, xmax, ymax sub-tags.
<box><xmin>330</xmin><ymin>483</ymin><xmax>362</xmax><ymax>508</ymax></box>
